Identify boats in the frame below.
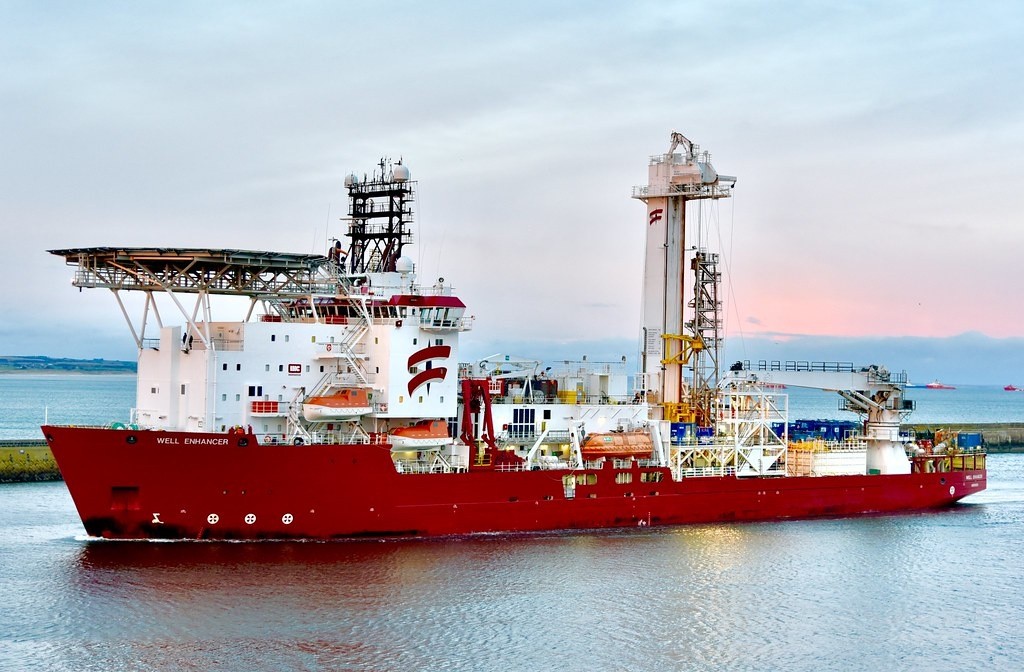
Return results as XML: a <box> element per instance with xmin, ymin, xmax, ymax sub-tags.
<box><xmin>1003</xmin><ymin>385</ymin><xmax>1024</xmax><ymax>392</ymax></box>
<box><xmin>302</xmin><ymin>387</ymin><xmax>373</xmax><ymax>421</ymax></box>
<box><xmin>579</xmin><ymin>427</ymin><xmax>655</xmax><ymax>460</ymax></box>
<box><xmin>386</xmin><ymin>419</ymin><xmax>455</xmax><ymax>452</ymax></box>
<box><xmin>904</xmin><ymin>379</ymin><xmax>957</xmax><ymax>389</ymax></box>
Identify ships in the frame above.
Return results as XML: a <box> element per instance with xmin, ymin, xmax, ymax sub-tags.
<box><xmin>32</xmin><ymin>126</ymin><xmax>994</xmax><ymax>542</ymax></box>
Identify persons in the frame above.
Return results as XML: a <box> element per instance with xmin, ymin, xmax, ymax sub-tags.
<box><xmin>229</xmin><ymin>425</ymin><xmax>245</xmax><ymax>434</ymax></box>
<box><xmin>248</xmin><ymin>424</ymin><xmax>252</xmax><ymax>434</ymax></box>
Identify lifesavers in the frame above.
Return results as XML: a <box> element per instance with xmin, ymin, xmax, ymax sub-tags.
<box><xmin>264</xmin><ymin>436</ymin><xmax>271</xmax><ymax>443</ymax></box>
<box><xmin>326</xmin><ymin>344</ymin><xmax>332</xmax><ymax>352</ymax></box>
